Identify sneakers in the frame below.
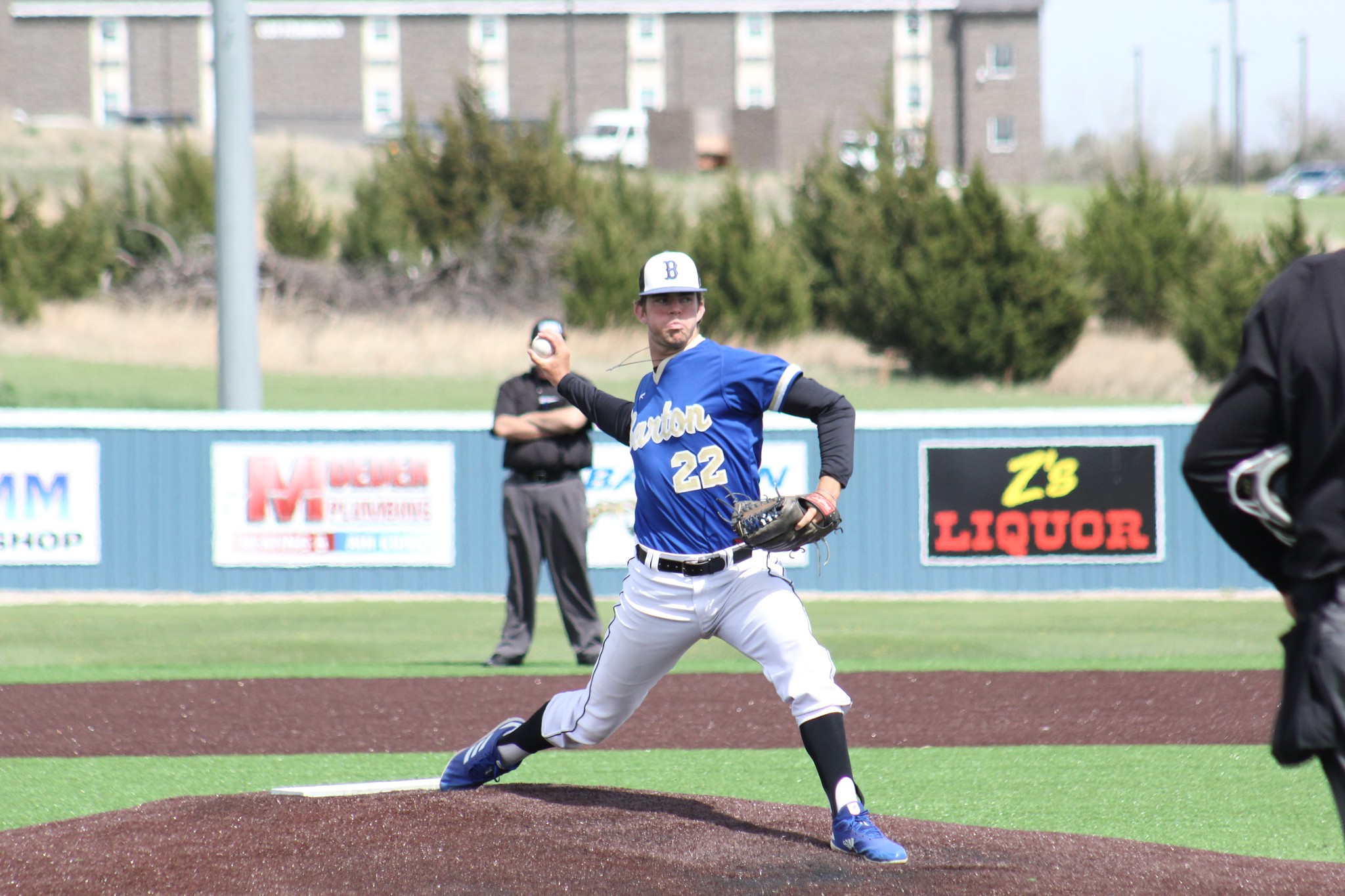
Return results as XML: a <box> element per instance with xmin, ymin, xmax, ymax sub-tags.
<box><xmin>830</xmin><ymin>801</ymin><xmax>908</xmax><ymax>864</ymax></box>
<box><xmin>439</xmin><ymin>717</ymin><xmax>527</xmax><ymax>792</ymax></box>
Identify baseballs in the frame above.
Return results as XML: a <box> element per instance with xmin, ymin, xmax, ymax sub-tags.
<box><xmin>532</xmin><ymin>335</ymin><xmax>553</xmax><ymax>358</ymax></box>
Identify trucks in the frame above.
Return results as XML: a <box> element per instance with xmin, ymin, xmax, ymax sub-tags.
<box><xmin>573</xmin><ymin>109</ymin><xmax>650</xmax><ymax>169</ymax></box>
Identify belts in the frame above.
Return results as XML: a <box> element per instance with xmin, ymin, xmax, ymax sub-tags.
<box><xmin>518</xmin><ymin>471</ymin><xmax>547</xmax><ymax>482</ymax></box>
<box><xmin>635</xmin><ymin>544</ymin><xmax>752</xmax><ymax>577</ymax></box>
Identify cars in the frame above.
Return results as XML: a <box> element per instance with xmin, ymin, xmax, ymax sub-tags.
<box><xmin>1266</xmin><ymin>157</ymin><xmax>1345</xmax><ymax>199</ymax></box>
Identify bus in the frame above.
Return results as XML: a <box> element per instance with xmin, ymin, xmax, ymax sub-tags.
<box><xmin>379</xmin><ymin>118</ymin><xmax>552</xmax><ymax>156</ymax></box>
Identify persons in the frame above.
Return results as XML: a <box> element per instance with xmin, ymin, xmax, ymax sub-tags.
<box><xmin>439</xmin><ymin>250</ymin><xmax>908</xmax><ymax>865</ymax></box>
<box><xmin>694</xmin><ymin>128</ymin><xmax>731</xmax><ymax>169</ymax></box>
<box><xmin>1182</xmin><ymin>248</ymin><xmax>1345</xmax><ymax>848</ymax></box>
<box><xmin>485</xmin><ymin>319</ymin><xmax>603</xmax><ymax>666</ymax></box>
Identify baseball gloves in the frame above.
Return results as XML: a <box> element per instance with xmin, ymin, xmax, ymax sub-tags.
<box><xmin>729</xmin><ymin>492</ymin><xmax>842</xmax><ymax>552</ymax></box>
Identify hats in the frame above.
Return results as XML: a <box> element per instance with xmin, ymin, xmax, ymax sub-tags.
<box><xmin>530</xmin><ymin>319</ymin><xmax>566</xmax><ymax>341</ymax></box>
<box><xmin>639</xmin><ymin>250</ymin><xmax>708</xmax><ymax>296</ymax></box>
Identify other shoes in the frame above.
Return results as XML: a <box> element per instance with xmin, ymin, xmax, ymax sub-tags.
<box><xmin>578</xmin><ymin>658</ymin><xmax>597</xmax><ymax>664</ymax></box>
<box><xmin>483</xmin><ymin>654</ymin><xmax>522</xmax><ymax>666</ymax></box>
